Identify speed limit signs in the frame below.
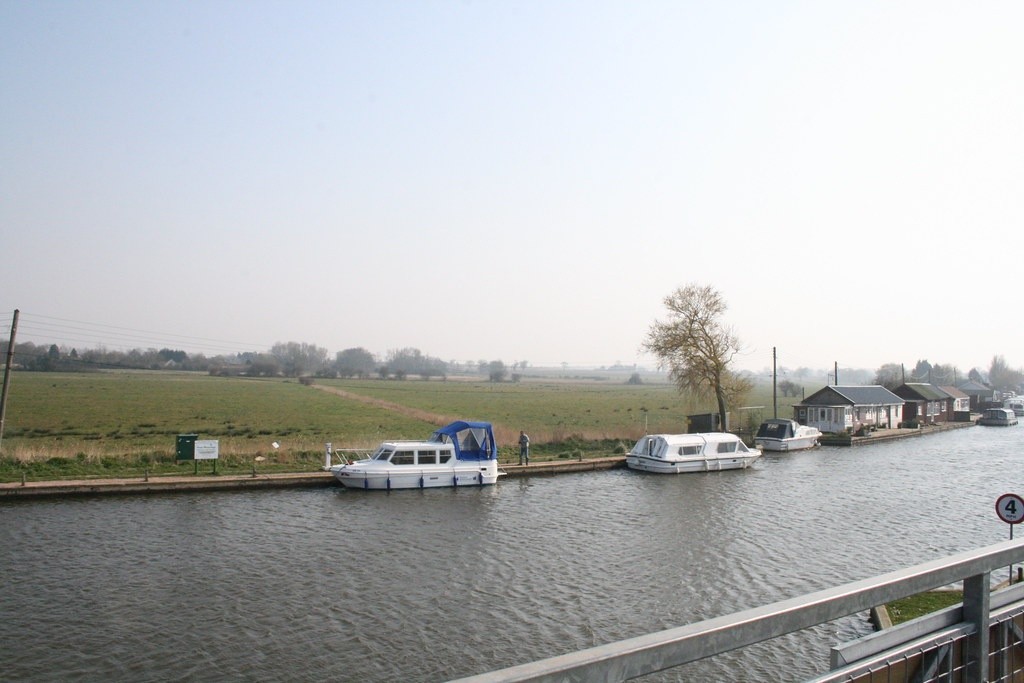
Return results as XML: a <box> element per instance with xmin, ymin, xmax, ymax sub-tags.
<box><xmin>996</xmin><ymin>493</ymin><xmax>1024</xmax><ymax>524</ymax></box>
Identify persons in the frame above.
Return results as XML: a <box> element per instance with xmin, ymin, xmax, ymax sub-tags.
<box><xmin>518</xmin><ymin>431</ymin><xmax>530</xmax><ymax>465</ymax></box>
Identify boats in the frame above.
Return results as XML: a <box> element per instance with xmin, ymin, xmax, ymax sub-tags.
<box><xmin>754</xmin><ymin>418</ymin><xmax>823</xmax><ymax>453</ymax></box>
<box><xmin>626</xmin><ymin>430</ymin><xmax>763</xmax><ymax>473</ymax></box>
<box><xmin>979</xmin><ymin>395</ymin><xmax>1024</xmax><ymax>427</ymax></box>
<box><xmin>328</xmin><ymin>420</ymin><xmax>507</xmax><ymax>487</ymax></box>
